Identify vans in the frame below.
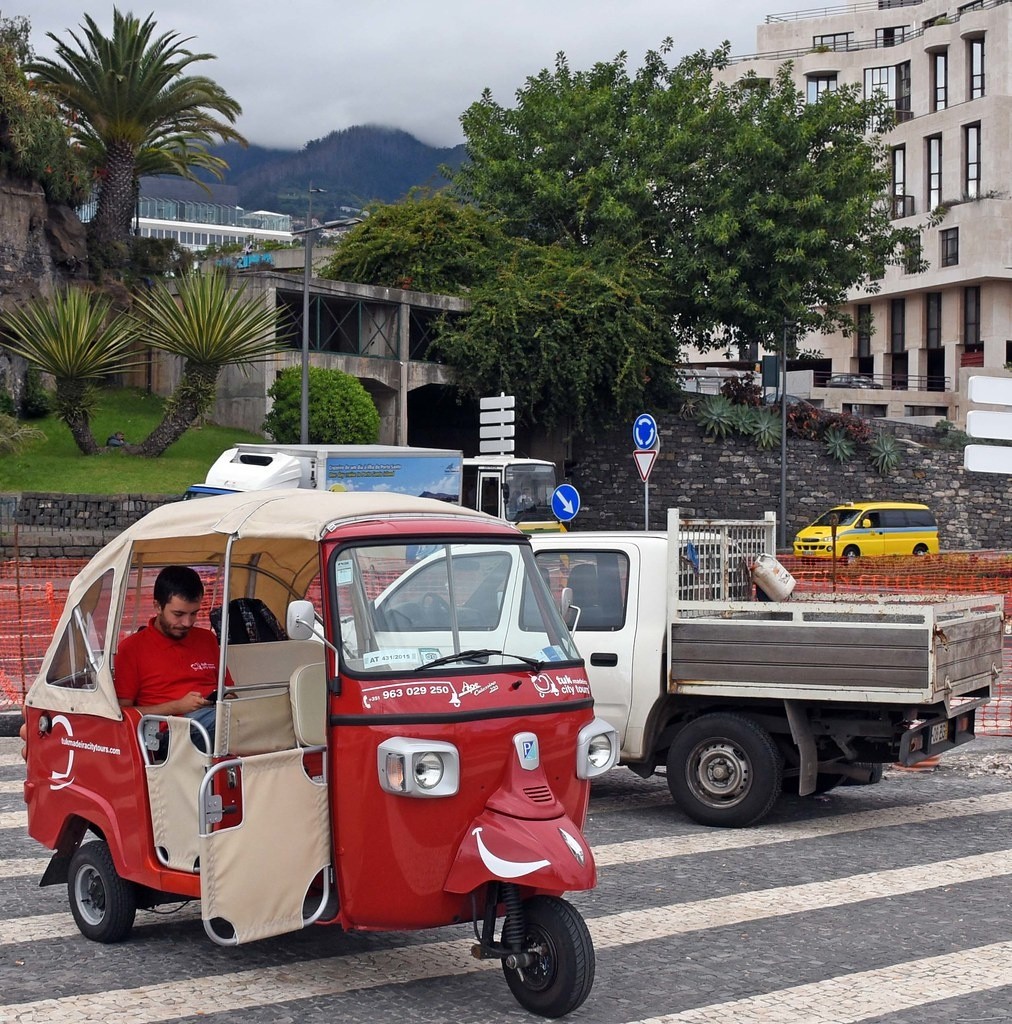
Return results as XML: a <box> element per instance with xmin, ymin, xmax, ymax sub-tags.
<box><xmin>792</xmin><ymin>502</ymin><xmax>940</xmax><ymax>562</ymax></box>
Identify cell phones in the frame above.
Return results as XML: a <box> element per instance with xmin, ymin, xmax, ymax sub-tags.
<box><xmin>206</xmin><ymin>689</ymin><xmax>218</xmax><ymax>705</ymax></box>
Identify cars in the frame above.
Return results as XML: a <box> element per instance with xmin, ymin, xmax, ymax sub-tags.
<box><xmin>824</xmin><ymin>373</ymin><xmax>884</xmax><ymax>389</ymax></box>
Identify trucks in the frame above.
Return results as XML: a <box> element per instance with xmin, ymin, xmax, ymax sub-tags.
<box><xmin>297</xmin><ymin>506</ymin><xmax>1007</xmax><ymax>830</ymax></box>
<box><xmin>179</xmin><ymin>442</ymin><xmax>464</xmax><ymax>513</ymax></box>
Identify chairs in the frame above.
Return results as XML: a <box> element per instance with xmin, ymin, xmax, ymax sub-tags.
<box><xmin>291</xmin><ymin>658</ymin><xmax>393</xmax><ymax>750</ymax></box>
<box><xmin>567</xmin><ymin>563</ymin><xmax>605</xmax><ymax>627</ymax></box>
<box><xmin>522</xmin><ymin>565</ymin><xmax>551</xmax><ymax>627</ymax></box>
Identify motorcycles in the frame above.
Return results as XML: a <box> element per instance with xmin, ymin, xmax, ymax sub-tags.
<box><xmin>20</xmin><ymin>484</ymin><xmax>623</xmax><ymax>1019</ymax></box>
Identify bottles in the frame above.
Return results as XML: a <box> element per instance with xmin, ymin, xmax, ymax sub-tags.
<box><xmin>750</xmin><ymin>553</ymin><xmax>797</xmax><ymax>602</ymax></box>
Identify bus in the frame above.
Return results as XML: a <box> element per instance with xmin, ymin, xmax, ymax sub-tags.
<box><xmin>462</xmin><ymin>456</ymin><xmax>573</xmax><ymax>567</ymax></box>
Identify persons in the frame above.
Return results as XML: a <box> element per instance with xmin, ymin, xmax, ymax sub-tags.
<box><xmin>517</xmin><ymin>488</ymin><xmax>532</xmax><ymax>506</ymax></box>
<box><xmin>107</xmin><ymin>431</ymin><xmax>130</xmax><ymax>447</ymax></box>
<box><xmin>116</xmin><ymin>565</ymin><xmax>239</xmax><ymax>750</ymax></box>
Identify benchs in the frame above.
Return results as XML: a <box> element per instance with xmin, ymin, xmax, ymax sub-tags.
<box><xmin>150</xmin><ymin>639</ymin><xmax>326</xmax><ymax>766</ymax></box>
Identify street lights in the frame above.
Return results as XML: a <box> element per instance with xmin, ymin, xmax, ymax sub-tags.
<box><xmin>291</xmin><ymin>178</ymin><xmax>366</xmax><ymax>446</ymax></box>
